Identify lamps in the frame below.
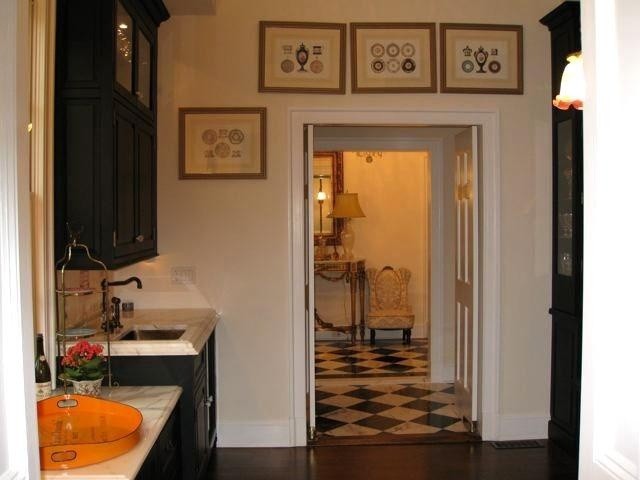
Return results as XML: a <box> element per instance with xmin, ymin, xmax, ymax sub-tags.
<box><xmin>327</xmin><ymin>194</ymin><xmax>367</xmax><ymax>260</ymax></box>
<box><xmin>551</xmin><ymin>54</ymin><xmax>583</xmax><ymax>112</ymax></box>
<box><xmin>314</xmin><ymin>174</ymin><xmax>331</xmax><ymax>236</ymax></box>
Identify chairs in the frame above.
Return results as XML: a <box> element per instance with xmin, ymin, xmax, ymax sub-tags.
<box><xmin>366</xmin><ymin>266</ymin><xmax>415</xmax><ymax>346</ymax></box>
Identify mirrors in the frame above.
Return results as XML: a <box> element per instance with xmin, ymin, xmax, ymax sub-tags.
<box><xmin>313</xmin><ymin>151</ymin><xmax>345</xmax><ymax>245</ymax></box>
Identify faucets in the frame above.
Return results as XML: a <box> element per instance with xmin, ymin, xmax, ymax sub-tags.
<box><xmin>101</xmin><ymin>276</ymin><xmax>142</xmax><ymax>333</ymax></box>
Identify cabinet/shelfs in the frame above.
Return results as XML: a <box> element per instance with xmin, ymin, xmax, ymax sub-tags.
<box><xmin>135</xmin><ymin>402</ymin><xmax>183</xmax><ymax>480</ymax></box>
<box><xmin>56</xmin><ymin>329</ymin><xmax>217</xmax><ymax>480</ymax></box>
<box><xmin>55</xmin><ymin>1</ymin><xmax>172</xmax><ymax>271</ymax></box>
<box><xmin>539</xmin><ymin>1</ymin><xmax>583</xmax><ymax>479</ymax></box>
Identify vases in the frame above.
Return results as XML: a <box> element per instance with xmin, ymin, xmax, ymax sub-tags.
<box><xmin>72</xmin><ymin>381</ymin><xmax>104</xmax><ymax>397</ymax></box>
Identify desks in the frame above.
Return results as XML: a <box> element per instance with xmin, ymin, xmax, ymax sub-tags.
<box><xmin>314</xmin><ymin>259</ymin><xmax>365</xmax><ymax>346</ymax></box>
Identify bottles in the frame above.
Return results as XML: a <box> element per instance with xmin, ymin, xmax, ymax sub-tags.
<box><xmin>35</xmin><ymin>333</ymin><xmax>51</xmax><ymax>402</ymax></box>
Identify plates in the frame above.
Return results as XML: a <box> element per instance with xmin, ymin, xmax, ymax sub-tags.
<box><xmin>57</xmin><ymin>328</ymin><xmax>98</xmax><ymax>338</ymax></box>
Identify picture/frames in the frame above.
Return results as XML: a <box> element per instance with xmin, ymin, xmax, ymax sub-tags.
<box><xmin>440</xmin><ymin>21</ymin><xmax>524</xmax><ymax>95</ymax></box>
<box><xmin>178</xmin><ymin>108</ymin><xmax>266</xmax><ymax>180</ymax></box>
<box><xmin>350</xmin><ymin>21</ymin><xmax>437</xmax><ymax>94</ymax></box>
<box><xmin>259</xmin><ymin>19</ymin><xmax>346</xmax><ymax>95</ymax></box>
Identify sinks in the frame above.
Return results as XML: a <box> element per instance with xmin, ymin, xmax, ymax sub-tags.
<box><xmin>114</xmin><ymin>324</ymin><xmax>187</xmax><ymax>342</ymax></box>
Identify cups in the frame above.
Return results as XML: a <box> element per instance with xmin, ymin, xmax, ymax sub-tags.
<box><xmin>122</xmin><ymin>298</ymin><xmax>134</xmax><ymax>318</ymax></box>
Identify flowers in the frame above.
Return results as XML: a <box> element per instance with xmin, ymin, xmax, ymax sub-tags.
<box><xmin>61</xmin><ymin>341</ymin><xmax>109</xmax><ymax>380</ymax></box>
